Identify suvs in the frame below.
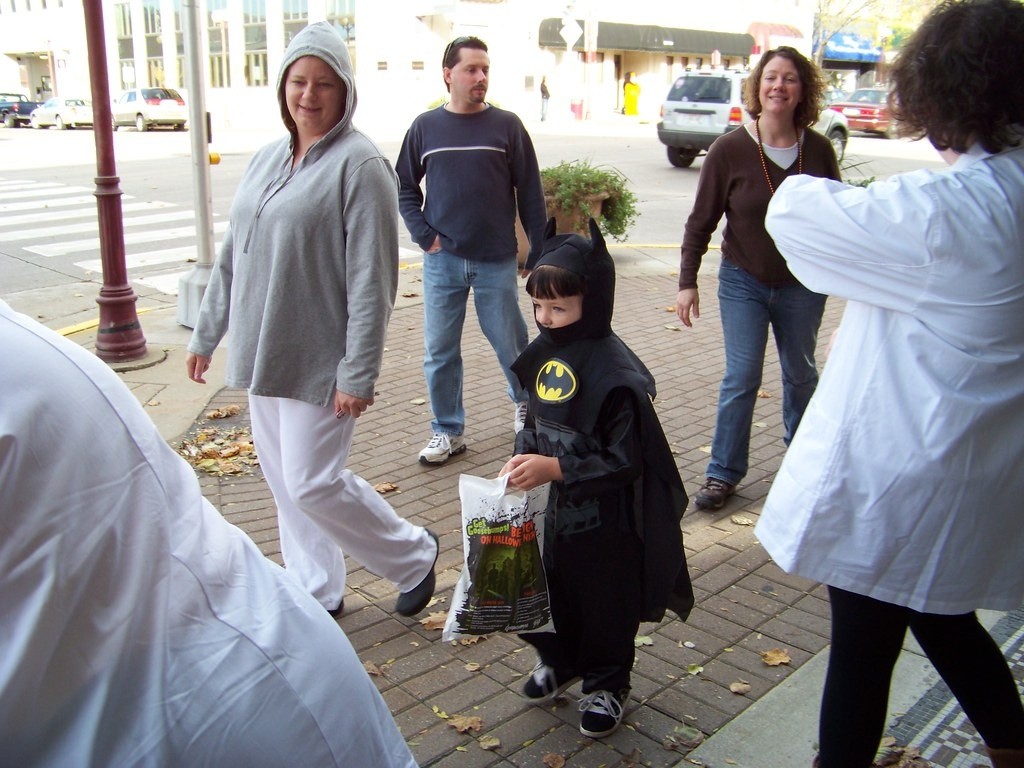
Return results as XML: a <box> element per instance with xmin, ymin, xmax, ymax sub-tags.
<box><xmin>653</xmin><ymin>65</ymin><xmax>850</xmax><ymax>166</ymax></box>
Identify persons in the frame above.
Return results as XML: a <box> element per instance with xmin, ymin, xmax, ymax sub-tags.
<box><xmin>507</xmin><ymin>216</ymin><xmax>696</xmax><ymax>737</ymax></box>
<box><xmin>740</xmin><ymin>0</ymin><xmax>1024</xmax><ymax>768</ymax></box>
<box><xmin>184</xmin><ymin>16</ymin><xmax>441</xmax><ymax>618</ymax></box>
<box><xmin>678</xmin><ymin>45</ymin><xmax>841</xmax><ymax>509</ymax></box>
<box><xmin>394</xmin><ymin>38</ymin><xmax>547</xmax><ymax>467</ymax></box>
<box><xmin>0</xmin><ymin>300</ymin><xmax>422</xmax><ymax>768</ymax></box>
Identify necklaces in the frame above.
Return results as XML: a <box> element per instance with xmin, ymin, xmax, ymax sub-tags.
<box><xmin>757</xmin><ymin>113</ymin><xmax>804</xmax><ymax>196</ymax></box>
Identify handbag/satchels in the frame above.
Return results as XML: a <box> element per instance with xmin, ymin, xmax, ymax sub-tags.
<box><xmin>441</xmin><ymin>474</ymin><xmax>557</xmax><ymax>642</ymax></box>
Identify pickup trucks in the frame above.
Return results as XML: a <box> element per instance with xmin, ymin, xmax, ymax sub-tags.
<box><xmin>0</xmin><ymin>93</ymin><xmax>46</xmax><ymax>129</ymax></box>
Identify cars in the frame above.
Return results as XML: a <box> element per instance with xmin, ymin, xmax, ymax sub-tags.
<box><xmin>829</xmin><ymin>87</ymin><xmax>909</xmax><ymax>140</ymax></box>
<box><xmin>111</xmin><ymin>88</ymin><xmax>188</xmax><ymax>132</ymax></box>
<box><xmin>28</xmin><ymin>96</ymin><xmax>94</xmax><ymax>130</ymax></box>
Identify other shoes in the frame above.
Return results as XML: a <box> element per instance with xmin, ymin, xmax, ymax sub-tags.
<box><xmin>326</xmin><ymin>597</ymin><xmax>344</xmax><ymax>616</ymax></box>
<box><xmin>395</xmin><ymin>527</ymin><xmax>439</xmax><ymax>617</ymax></box>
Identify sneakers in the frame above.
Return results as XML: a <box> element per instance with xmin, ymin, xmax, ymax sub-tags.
<box><xmin>418</xmin><ymin>432</ymin><xmax>466</xmax><ymax>466</ymax></box>
<box><xmin>695</xmin><ymin>476</ymin><xmax>736</xmax><ymax>510</ymax></box>
<box><xmin>521</xmin><ymin>660</ymin><xmax>582</xmax><ymax>705</ymax></box>
<box><xmin>576</xmin><ymin>685</ymin><xmax>631</xmax><ymax>738</ymax></box>
<box><xmin>513</xmin><ymin>400</ymin><xmax>528</xmax><ymax>435</ymax></box>
